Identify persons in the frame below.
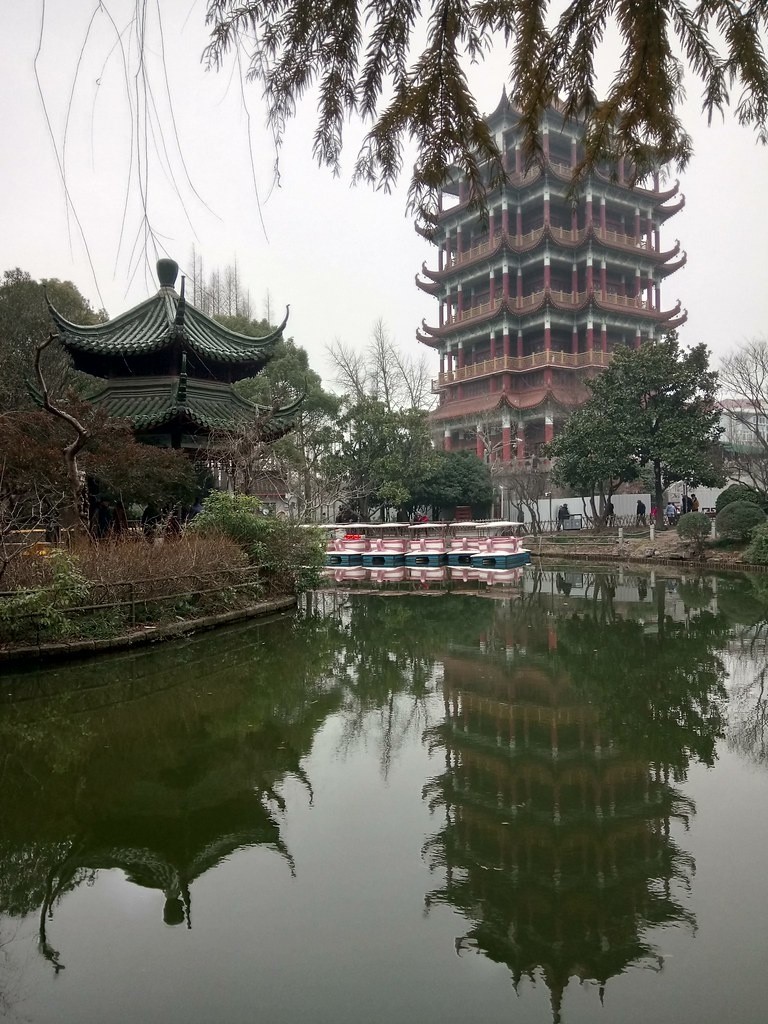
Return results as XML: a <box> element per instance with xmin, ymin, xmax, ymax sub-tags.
<box><xmin>45</xmin><ymin>517</ymin><xmax>59</xmax><ymax>542</ymax></box>
<box><xmin>606</xmin><ymin>499</ymin><xmax>614</xmax><ymax>525</ymax></box>
<box><xmin>652</xmin><ymin>506</ymin><xmax>657</xmax><ymax>519</ymax></box>
<box><xmin>681</xmin><ymin>493</ymin><xmax>699</xmax><ymax>514</ymax></box>
<box><xmin>636</xmin><ymin>500</ymin><xmax>646</xmax><ymax>525</ymax></box>
<box><xmin>192</xmin><ymin>497</ymin><xmax>202</xmax><ymax>515</ymax></box>
<box><xmin>414</xmin><ymin>511</ymin><xmax>428</xmax><ymax>536</ymax></box>
<box><xmin>666</xmin><ymin>502</ymin><xmax>681</xmax><ymax>525</ymax></box>
<box><xmin>557</xmin><ymin>503</ymin><xmax>569</xmax><ymax>531</ymax></box>
<box><xmin>142</xmin><ymin>501</ymin><xmax>161</xmax><ymax>536</ymax></box>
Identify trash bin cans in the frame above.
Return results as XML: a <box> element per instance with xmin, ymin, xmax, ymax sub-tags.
<box><xmin>562</xmin><ymin>514</ymin><xmax>582</xmax><ymax>531</ymax></box>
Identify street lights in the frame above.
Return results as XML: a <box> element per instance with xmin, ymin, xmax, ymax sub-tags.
<box><xmin>498</xmin><ymin>486</ymin><xmax>503</xmax><ymax>519</ymax></box>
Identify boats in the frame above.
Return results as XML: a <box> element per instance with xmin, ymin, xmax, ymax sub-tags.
<box><xmin>404</xmin><ymin>523</ymin><xmax>452</xmax><ymax>567</ymax></box>
<box><xmin>292</xmin><ymin>523</ymin><xmax>370</xmax><ymax>567</ymax></box>
<box><xmin>470</xmin><ymin>521</ymin><xmax>532</xmax><ymax>569</ymax></box>
<box><xmin>362</xmin><ymin>523</ymin><xmax>410</xmax><ymax>568</ymax></box>
<box><xmin>309</xmin><ymin>567</ymin><xmax>528</xmax><ymax>588</ymax></box>
<box><xmin>447</xmin><ymin>521</ymin><xmax>480</xmax><ymax>566</ymax></box>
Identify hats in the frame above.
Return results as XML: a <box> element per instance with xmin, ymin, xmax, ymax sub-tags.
<box><xmin>637</xmin><ymin>500</ymin><xmax>641</xmax><ymax>503</ymax></box>
